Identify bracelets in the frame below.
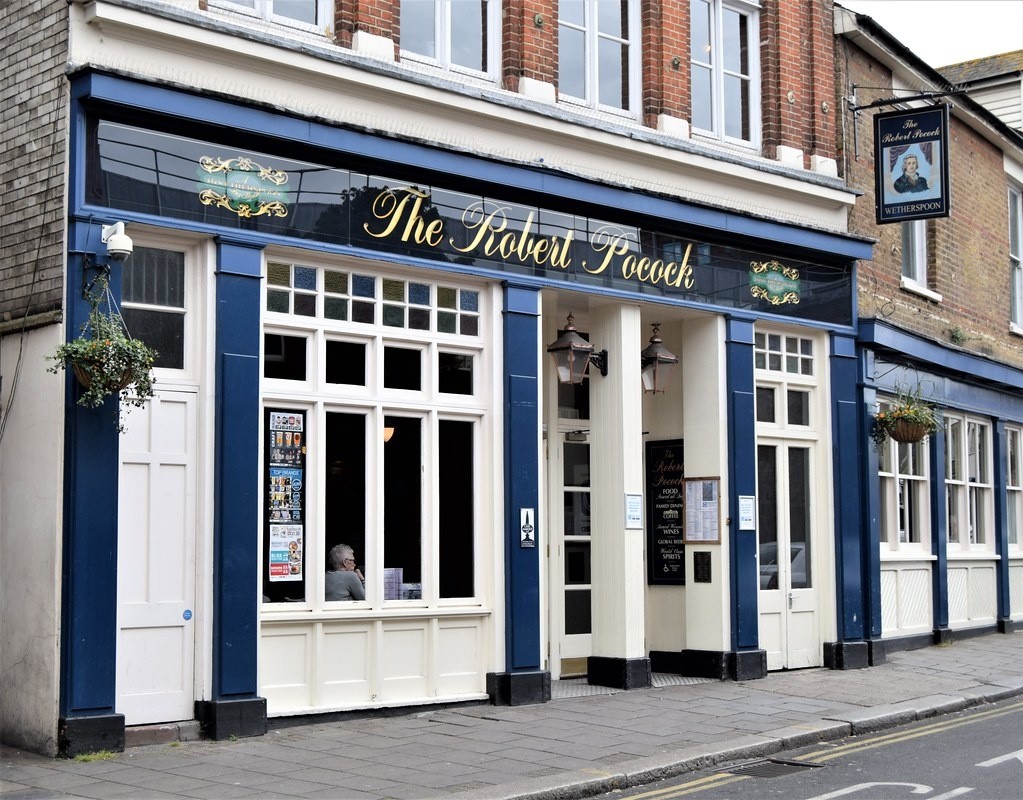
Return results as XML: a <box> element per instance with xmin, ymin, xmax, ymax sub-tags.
<box><xmin>362</xmin><ymin>582</ymin><xmax>365</xmax><ymax>584</ymax></box>
<box><xmin>361</xmin><ymin>579</ymin><xmax>365</xmax><ymax>581</ymax></box>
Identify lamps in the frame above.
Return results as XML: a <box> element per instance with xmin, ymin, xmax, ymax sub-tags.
<box><xmin>548</xmin><ymin>311</ymin><xmax>608</xmax><ymax>383</ymax></box>
<box><xmin>640</xmin><ymin>323</ymin><xmax>678</xmax><ymax>395</ymax></box>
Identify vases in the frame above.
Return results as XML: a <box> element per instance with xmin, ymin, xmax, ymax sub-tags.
<box><xmin>71</xmin><ymin>358</ymin><xmax>134</xmax><ymax>394</ymax></box>
<box><xmin>885</xmin><ymin>417</ymin><xmax>931</xmax><ymax>444</ymax></box>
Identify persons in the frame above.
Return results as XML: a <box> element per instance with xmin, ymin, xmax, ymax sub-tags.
<box><xmin>325</xmin><ymin>544</ymin><xmax>365</xmax><ymax>601</ymax></box>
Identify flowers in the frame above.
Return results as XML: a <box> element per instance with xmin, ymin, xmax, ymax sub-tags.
<box><xmin>45</xmin><ymin>278</ymin><xmax>160</xmax><ymax>437</ymax></box>
<box><xmin>873</xmin><ymin>367</ymin><xmax>946</xmax><ymax>452</ymax></box>
